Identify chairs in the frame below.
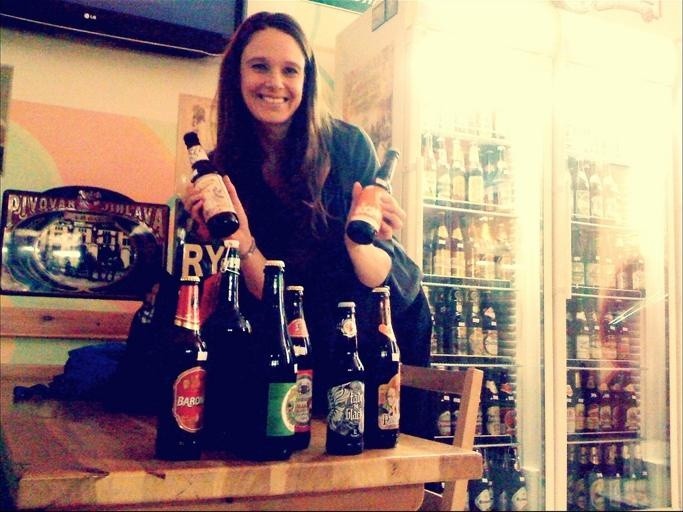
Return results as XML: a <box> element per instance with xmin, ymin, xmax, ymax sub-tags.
<box><xmin>396</xmin><ymin>360</ymin><xmax>485</xmax><ymax>511</ymax></box>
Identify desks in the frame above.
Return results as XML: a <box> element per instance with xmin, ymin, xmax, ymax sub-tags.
<box><xmin>0</xmin><ymin>361</ymin><xmax>485</xmax><ymax>511</ymax></box>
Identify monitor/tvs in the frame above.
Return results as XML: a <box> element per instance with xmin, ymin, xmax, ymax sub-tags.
<box><xmin>0</xmin><ymin>0</ymin><xmax>250</xmax><ymax>59</ymax></box>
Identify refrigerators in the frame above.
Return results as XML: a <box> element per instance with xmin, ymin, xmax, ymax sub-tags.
<box><xmin>328</xmin><ymin>1</ymin><xmax>682</xmax><ymax>511</ymax></box>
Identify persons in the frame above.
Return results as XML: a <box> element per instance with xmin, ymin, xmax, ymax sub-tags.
<box><xmin>181</xmin><ymin>12</ymin><xmax>435</xmax><ymax>445</ymax></box>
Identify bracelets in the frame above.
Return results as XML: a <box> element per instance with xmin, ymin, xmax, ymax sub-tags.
<box><xmin>233</xmin><ymin>237</ymin><xmax>257</xmax><ymax>261</ymax></box>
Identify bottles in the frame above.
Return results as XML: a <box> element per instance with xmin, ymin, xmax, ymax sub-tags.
<box><xmin>427</xmin><ymin>139</ymin><xmax>646</xmax><ymax>506</ymax></box>
<box><xmin>182</xmin><ymin>130</ymin><xmax>240</xmax><ymax>239</ymax></box>
<box><xmin>344</xmin><ymin>150</ymin><xmax>402</xmax><ymax>245</ymax></box>
<box><xmin>326</xmin><ymin>300</ymin><xmax>366</xmax><ymax>454</ymax></box>
<box><xmin>157</xmin><ymin>245</ymin><xmax>312</xmax><ymax>460</ymax></box>
<box><xmin>368</xmin><ymin>286</ymin><xmax>401</xmax><ymax>445</ymax></box>
